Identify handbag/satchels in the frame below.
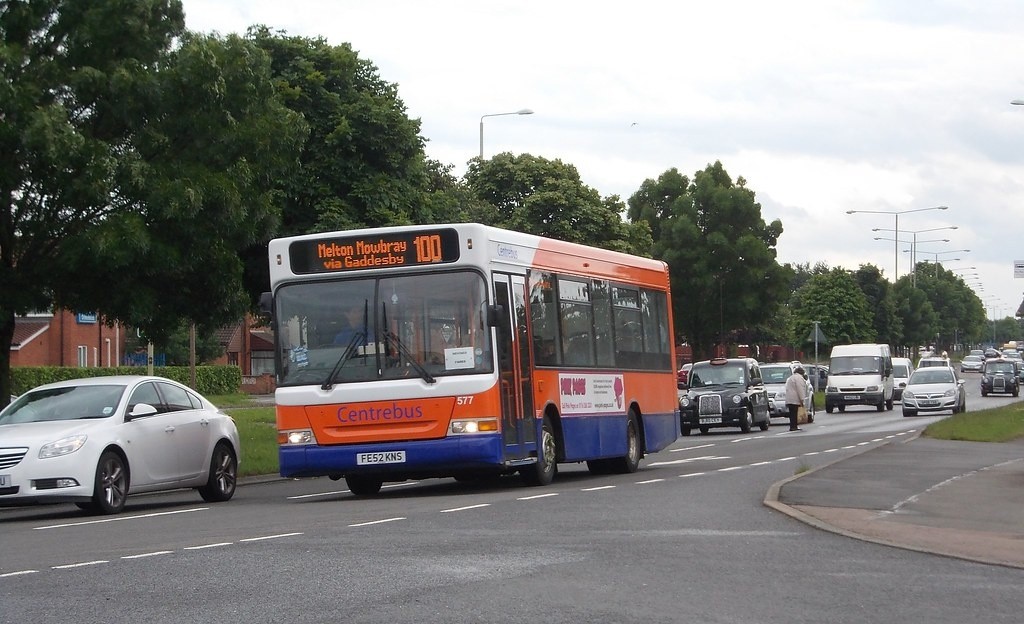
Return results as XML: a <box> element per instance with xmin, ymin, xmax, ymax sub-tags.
<box><xmin>797</xmin><ymin>402</ymin><xmax>808</xmax><ymax>424</ymax></box>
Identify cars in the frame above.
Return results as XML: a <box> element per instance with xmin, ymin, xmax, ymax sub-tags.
<box><xmin>960</xmin><ymin>356</ymin><xmax>985</xmax><ymax>373</ymax></box>
<box><xmin>1003</xmin><ymin>344</ymin><xmax>1024</xmax><ymax>384</ymax></box>
<box><xmin>676</xmin><ymin>364</ymin><xmax>692</xmax><ymax>391</ymax></box>
<box><xmin>917</xmin><ymin>356</ymin><xmax>950</xmax><ymax>368</ymax></box>
<box><xmin>0</xmin><ymin>375</ymin><xmax>241</xmax><ymax>514</ymax></box>
<box><xmin>802</xmin><ymin>364</ymin><xmax>829</xmax><ymax>389</ymax></box>
<box><xmin>679</xmin><ymin>358</ymin><xmax>771</xmax><ymax>436</ymax></box>
<box><xmin>969</xmin><ymin>350</ymin><xmax>986</xmax><ymax>361</ymax></box>
<box><xmin>899</xmin><ymin>367</ymin><xmax>966</xmax><ymax>417</ymax></box>
<box><xmin>985</xmin><ymin>349</ymin><xmax>996</xmax><ymax>357</ymax></box>
<box><xmin>978</xmin><ymin>359</ymin><xmax>1021</xmax><ymax>397</ymax></box>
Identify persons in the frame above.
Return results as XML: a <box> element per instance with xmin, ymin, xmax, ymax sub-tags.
<box><xmin>785</xmin><ymin>367</ymin><xmax>807</xmax><ymax>432</ymax></box>
<box><xmin>330</xmin><ymin>296</ymin><xmax>394</xmax><ymax>383</ymax></box>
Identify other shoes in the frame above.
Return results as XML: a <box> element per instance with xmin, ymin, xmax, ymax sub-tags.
<box><xmin>791</xmin><ymin>428</ymin><xmax>802</xmax><ymax>431</ymax></box>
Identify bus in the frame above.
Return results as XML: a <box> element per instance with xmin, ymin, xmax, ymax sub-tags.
<box><xmin>260</xmin><ymin>223</ymin><xmax>681</xmax><ymax>496</ymax></box>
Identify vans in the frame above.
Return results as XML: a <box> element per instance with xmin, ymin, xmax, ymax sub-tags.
<box><xmin>825</xmin><ymin>344</ymin><xmax>894</xmax><ymax>413</ymax></box>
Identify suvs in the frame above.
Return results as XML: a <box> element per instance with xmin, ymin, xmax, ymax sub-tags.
<box><xmin>757</xmin><ymin>361</ymin><xmax>815</xmax><ymax>423</ymax></box>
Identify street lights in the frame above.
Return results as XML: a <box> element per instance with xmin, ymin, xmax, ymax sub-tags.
<box><xmin>979</xmin><ymin>295</ymin><xmax>1012</xmax><ymax>342</ymax></box>
<box><xmin>846</xmin><ymin>206</ymin><xmax>948</xmax><ymax>282</ymax></box>
<box><xmin>872</xmin><ymin>226</ymin><xmax>958</xmax><ymax>288</ymax></box>
<box><xmin>924</xmin><ymin>259</ymin><xmax>984</xmax><ymax>291</ymax></box>
<box><xmin>903</xmin><ymin>249</ymin><xmax>970</xmax><ymax>278</ymax></box>
<box><xmin>873</xmin><ymin>237</ymin><xmax>950</xmax><ymax>288</ymax></box>
<box><xmin>480</xmin><ymin>109</ymin><xmax>534</xmax><ymax>159</ymax></box>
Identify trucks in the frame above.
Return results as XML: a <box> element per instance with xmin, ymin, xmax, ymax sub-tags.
<box><xmin>891</xmin><ymin>358</ymin><xmax>914</xmax><ymax>400</ymax></box>
